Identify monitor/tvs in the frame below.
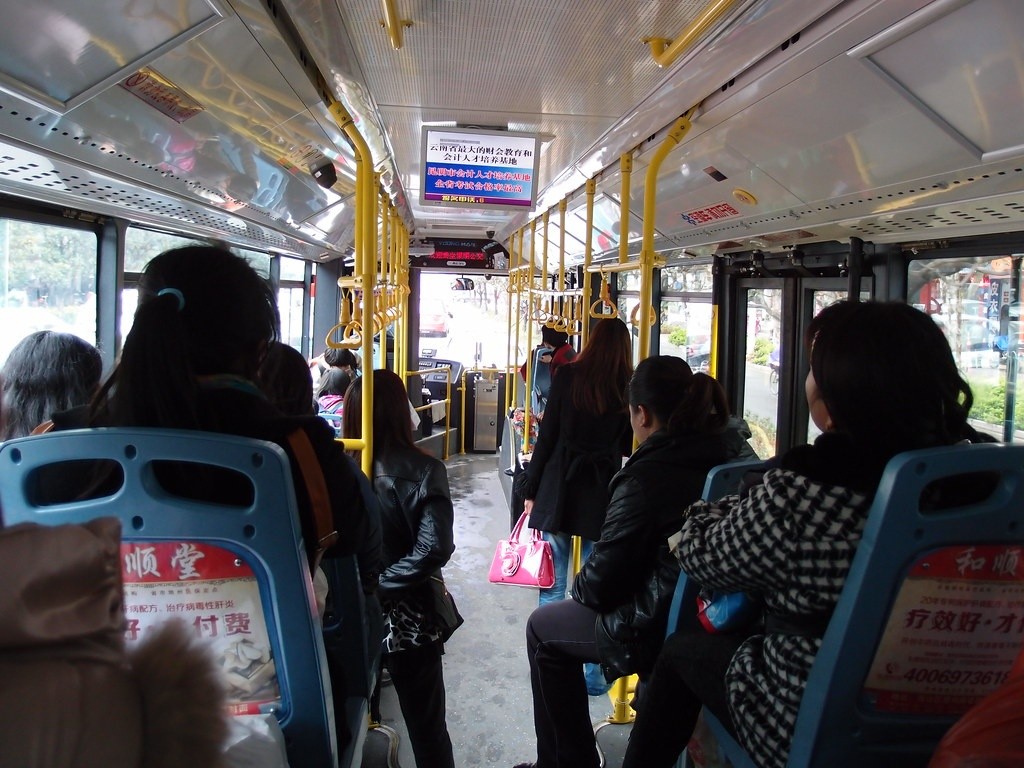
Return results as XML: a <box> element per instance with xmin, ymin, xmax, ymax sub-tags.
<box><xmin>418</xmin><ymin>126</ymin><xmax>541</xmax><ymax>212</ymax></box>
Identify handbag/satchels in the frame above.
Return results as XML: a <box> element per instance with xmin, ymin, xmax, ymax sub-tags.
<box><xmin>488</xmin><ymin>510</ymin><xmax>556</xmax><ymax>589</ymax></box>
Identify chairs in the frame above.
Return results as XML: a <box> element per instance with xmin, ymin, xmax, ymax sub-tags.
<box><xmin>353</xmin><ymin>343</ymin><xmax>382</xmax><ymax>377</ymax></box>
<box><xmin>522</xmin><ymin>345</ymin><xmax>553</xmax><ymax>435</ymax></box>
<box><xmin>318</xmin><ymin>413</ymin><xmax>342</xmax><ymax>438</ymax></box>
<box><xmin>636</xmin><ymin>442</ymin><xmax>1023</xmax><ymax>768</ymax></box>
<box><xmin>0</xmin><ymin>427</ymin><xmax>385</xmax><ymax>767</ymax></box>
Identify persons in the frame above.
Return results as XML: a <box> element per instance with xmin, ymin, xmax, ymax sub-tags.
<box><xmin>73</xmin><ymin>92</ymin><xmax>261</xmax><ymax>212</ymax></box>
<box><xmin>512</xmin><ymin>355</ymin><xmax>760</xmax><ymax>768</ymax></box>
<box><xmin>0</xmin><ymin>244</ymin><xmax>464</xmax><ymax>768</ymax></box>
<box><xmin>503</xmin><ymin>318</ymin><xmax>635</xmax><ymax>696</ymax></box>
<box><xmin>622</xmin><ymin>298</ymin><xmax>1001</xmax><ymax>768</ymax></box>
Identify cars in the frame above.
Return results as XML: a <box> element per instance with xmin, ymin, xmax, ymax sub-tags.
<box><xmin>913</xmin><ymin>297</ymin><xmax>1024</xmax><ymax>369</ymax></box>
<box><xmin>420</xmin><ymin>298</ymin><xmax>453</xmax><ymax>338</ymax></box>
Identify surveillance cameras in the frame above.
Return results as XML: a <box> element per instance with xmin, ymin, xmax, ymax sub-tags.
<box><xmin>486</xmin><ymin>230</ymin><xmax>495</xmax><ymax>240</ymax></box>
<box><xmin>309</xmin><ymin>158</ymin><xmax>338</xmax><ymax>189</ymax></box>
